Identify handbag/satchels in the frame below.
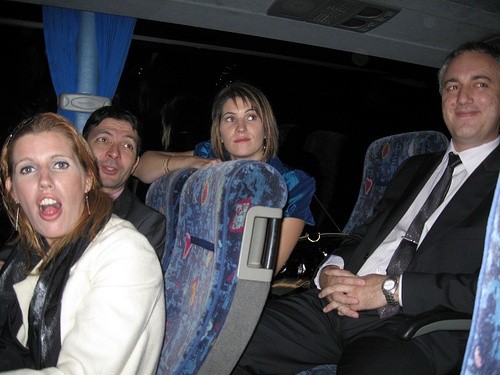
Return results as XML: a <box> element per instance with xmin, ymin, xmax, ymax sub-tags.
<box><xmin>272</xmin><ymin>194</ymin><xmax>349</xmax><ymax>299</ymax></box>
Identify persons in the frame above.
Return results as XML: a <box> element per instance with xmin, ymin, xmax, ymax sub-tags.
<box><xmin>133</xmin><ymin>82</ymin><xmax>316</xmax><ymax>276</ymax></box>
<box><xmin>0</xmin><ymin>111</ymin><xmax>166</xmax><ymax>372</ymax></box>
<box><xmin>82</xmin><ymin>104</ymin><xmax>165</xmax><ymax>266</ymax></box>
<box><xmin>160</xmin><ymin>102</ymin><xmax>178</xmax><ymax>151</ymax></box>
<box><xmin>234</xmin><ymin>42</ymin><xmax>499</xmax><ymax>372</ymax></box>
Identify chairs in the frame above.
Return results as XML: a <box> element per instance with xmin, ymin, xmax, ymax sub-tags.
<box><xmin>460</xmin><ymin>173</ymin><xmax>500</xmax><ymax>375</ymax></box>
<box><xmin>145</xmin><ymin>166</ymin><xmax>199</xmax><ymax>276</ymax></box>
<box><xmin>156</xmin><ymin>159</ymin><xmax>287</xmax><ymax>375</ymax></box>
<box><xmin>342</xmin><ymin>131</ymin><xmax>449</xmax><ymax>234</ymax></box>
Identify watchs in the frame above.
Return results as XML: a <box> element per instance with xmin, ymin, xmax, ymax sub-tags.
<box><xmin>381</xmin><ymin>273</ymin><xmax>400</xmax><ymax>306</ymax></box>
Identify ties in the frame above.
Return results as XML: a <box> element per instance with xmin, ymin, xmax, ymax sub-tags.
<box><xmin>377</xmin><ymin>152</ymin><xmax>462</xmax><ymax>319</ymax></box>
